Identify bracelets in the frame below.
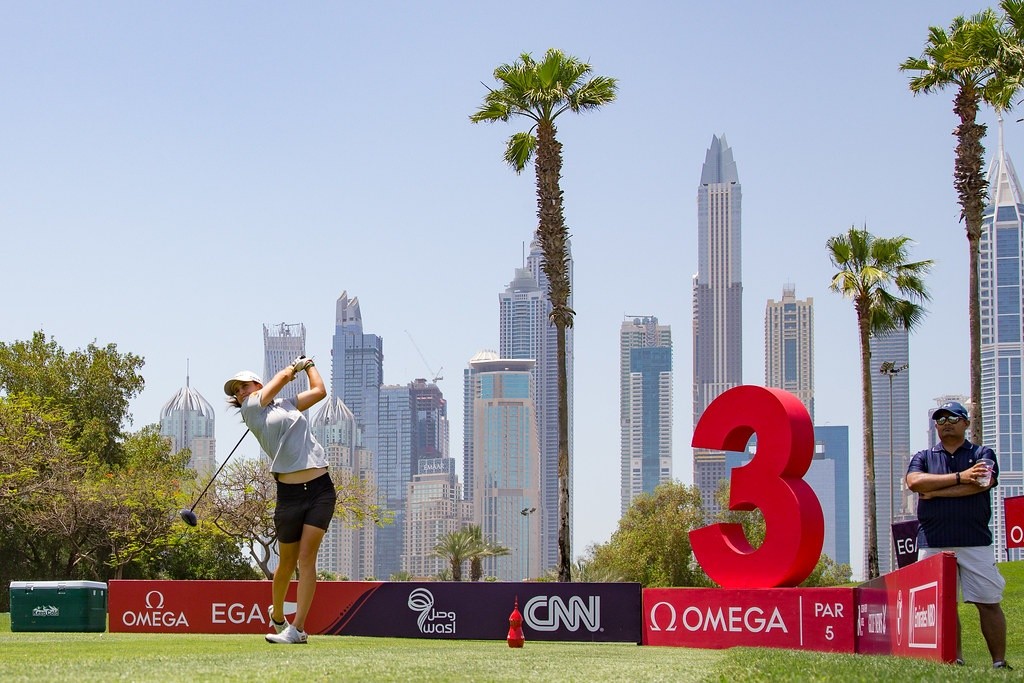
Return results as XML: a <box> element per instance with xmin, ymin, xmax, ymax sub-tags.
<box><xmin>956</xmin><ymin>472</ymin><xmax>960</xmax><ymax>484</ymax></box>
<box><xmin>305</xmin><ymin>360</ymin><xmax>314</xmax><ymax>370</ymax></box>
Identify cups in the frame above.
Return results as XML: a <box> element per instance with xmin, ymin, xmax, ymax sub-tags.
<box><xmin>976</xmin><ymin>458</ymin><xmax>995</xmax><ymax>487</ymax></box>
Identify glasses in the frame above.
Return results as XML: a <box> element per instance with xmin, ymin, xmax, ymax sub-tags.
<box><xmin>935</xmin><ymin>415</ymin><xmax>966</xmax><ymax>425</ymax></box>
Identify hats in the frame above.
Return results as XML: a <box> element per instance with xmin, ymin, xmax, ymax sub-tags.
<box><xmin>932</xmin><ymin>402</ymin><xmax>968</xmax><ymax>420</ymax></box>
<box><xmin>224</xmin><ymin>372</ymin><xmax>264</xmax><ymax>396</ymax></box>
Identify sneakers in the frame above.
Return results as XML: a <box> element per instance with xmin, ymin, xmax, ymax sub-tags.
<box><xmin>267</xmin><ymin>605</ymin><xmax>291</xmax><ymax>634</ymax></box>
<box><xmin>265</xmin><ymin>625</ymin><xmax>308</xmax><ymax>644</ymax></box>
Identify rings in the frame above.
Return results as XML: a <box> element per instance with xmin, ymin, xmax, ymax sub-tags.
<box><xmin>971</xmin><ymin>478</ymin><xmax>975</xmax><ymax>483</ymax></box>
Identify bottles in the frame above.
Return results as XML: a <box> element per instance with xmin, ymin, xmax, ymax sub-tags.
<box><xmin>507</xmin><ymin>595</ymin><xmax>525</xmax><ymax>648</ymax></box>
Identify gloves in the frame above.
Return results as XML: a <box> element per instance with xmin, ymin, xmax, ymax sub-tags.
<box><xmin>290</xmin><ymin>355</ymin><xmax>313</xmax><ymax>372</ymax></box>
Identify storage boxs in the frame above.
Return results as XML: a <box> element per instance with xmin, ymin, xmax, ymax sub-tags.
<box><xmin>8</xmin><ymin>580</ymin><xmax>108</xmax><ymax>633</ymax></box>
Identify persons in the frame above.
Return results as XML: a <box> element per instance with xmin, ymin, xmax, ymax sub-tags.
<box><xmin>905</xmin><ymin>402</ymin><xmax>1014</xmax><ymax>670</ymax></box>
<box><xmin>224</xmin><ymin>356</ymin><xmax>336</xmax><ymax>644</ymax></box>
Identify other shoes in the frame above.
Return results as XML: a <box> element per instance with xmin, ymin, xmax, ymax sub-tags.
<box><xmin>994</xmin><ymin>661</ymin><xmax>1014</xmax><ymax>672</ymax></box>
<box><xmin>956</xmin><ymin>659</ymin><xmax>964</xmax><ymax>666</ymax></box>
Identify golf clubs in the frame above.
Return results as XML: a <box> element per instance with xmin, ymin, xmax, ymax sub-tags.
<box><xmin>179</xmin><ymin>353</ymin><xmax>309</xmax><ymax>527</ymax></box>
